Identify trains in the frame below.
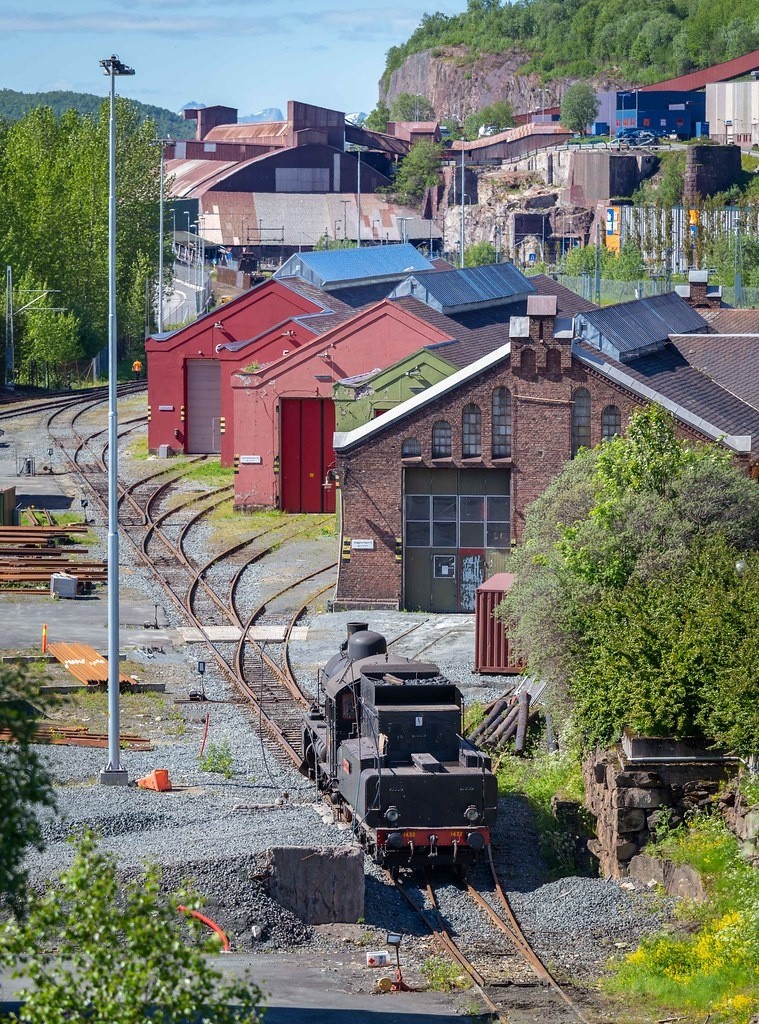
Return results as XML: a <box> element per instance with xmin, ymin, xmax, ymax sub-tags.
<box><xmin>298</xmin><ymin>621</ymin><xmax>500</xmax><ymax>869</ymax></box>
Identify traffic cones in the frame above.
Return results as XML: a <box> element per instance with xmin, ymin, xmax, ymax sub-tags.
<box><xmin>135</xmin><ymin>769</ymin><xmax>172</xmax><ymax>791</ymax></box>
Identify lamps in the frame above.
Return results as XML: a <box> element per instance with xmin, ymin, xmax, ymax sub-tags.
<box><xmin>386</xmin><ymin>933</ymin><xmax>402</xmax><ymax>946</ymax></box>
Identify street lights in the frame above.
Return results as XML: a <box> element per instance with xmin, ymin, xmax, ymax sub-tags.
<box><xmin>335</xmin><ymin>220</ymin><xmax>341</xmax><ymax>241</ymax></box>
<box><xmin>195</xmin><ymin>221</ymin><xmax>201</xmax><ymax>287</ymax></box>
<box><xmin>184</xmin><ymin>211</ymin><xmax>191</xmax><ymax>284</ymax></box>
<box><xmin>190</xmin><ymin>225</ymin><xmax>197</xmax><ymax>286</ymax></box>
<box><xmin>100</xmin><ymin>55</ymin><xmax>137</xmax><ymax>783</ymax></box>
<box><xmin>200</xmin><ymin>217</ymin><xmax>205</xmax><ymax>288</ymax></box>
<box><xmin>397</xmin><ymin>217</ymin><xmax>414</xmax><ymax>244</ymax></box>
<box><xmin>372</xmin><ymin>220</ymin><xmax>379</xmax><ymax>241</ymax></box>
<box><xmin>539</xmin><ymin>89</ymin><xmax>544</xmax><ymax>123</ymax></box>
<box><xmin>349</xmin><ymin>145</ymin><xmax>369</xmax><ymax>247</ymax></box>
<box><xmin>170</xmin><ymin>209</ymin><xmax>176</xmax><ymax>278</ymax></box>
<box><xmin>340</xmin><ymin>200</ymin><xmax>351</xmax><ymax>239</ymax></box>
<box><xmin>461</xmin><ymin>137</ymin><xmax>466</xmax><ymax>267</ymax></box>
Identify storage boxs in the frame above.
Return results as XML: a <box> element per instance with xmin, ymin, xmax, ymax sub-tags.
<box><xmin>474</xmin><ymin>572</ymin><xmax>541</xmax><ymax>676</ymax></box>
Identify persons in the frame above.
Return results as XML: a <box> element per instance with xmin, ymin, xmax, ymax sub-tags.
<box><xmin>133</xmin><ymin>358</ymin><xmax>143</xmax><ymax>382</ymax></box>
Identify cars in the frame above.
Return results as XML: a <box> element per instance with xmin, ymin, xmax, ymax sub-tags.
<box><xmin>440</xmin><ymin>126</ymin><xmax>449</xmax><ymax>138</ymax></box>
<box><xmin>616</xmin><ymin>127</ymin><xmax>670</xmax><ymax>140</ymax></box>
